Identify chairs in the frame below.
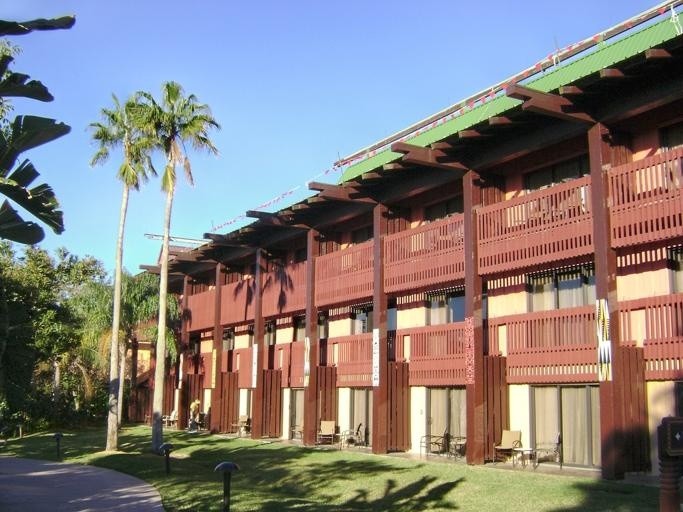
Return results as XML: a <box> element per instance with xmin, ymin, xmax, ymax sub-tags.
<box><xmin>418</xmin><ymin>426</ymin><xmax>467</xmax><ymax>461</ymax></box>
<box><xmin>316</xmin><ymin>420</ymin><xmax>363</xmax><ymax>451</ymax></box>
<box><xmin>491</xmin><ymin>429</ymin><xmax>563</xmax><ymax>472</ymax></box>
